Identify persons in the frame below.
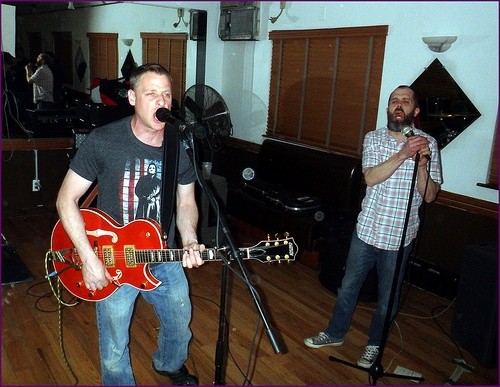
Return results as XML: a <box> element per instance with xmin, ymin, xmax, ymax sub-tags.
<box><xmin>1</xmin><ymin>50</ymin><xmax>30</xmax><ymax>206</ymax></box>
<box><xmin>135</xmin><ymin>163</ymin><xmax>163</xmax><ymax>224</ymax></box>
<box><xmin>56</xmin><ymin>63</ymin><xmax>204</xmax><ymax>387</ymax></box>
<box><xmin>25</xmin><ymin>53</ymin><xmax>54</xmax><ymax>123</ymax></box>
<box><xmin>303</xmin><ymin>85</ymin><xmax>443</xmax><ymax>369</ymax></box>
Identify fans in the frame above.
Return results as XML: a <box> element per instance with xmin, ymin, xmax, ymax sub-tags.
<box><xmin>179</xmin><ymin>83</ymin><xmax>231</xmax><ymax>250</ymax></box>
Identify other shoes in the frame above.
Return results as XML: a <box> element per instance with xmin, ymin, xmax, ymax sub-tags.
<box><xmin>152</xmin><ymin>362</ymin><xmax>198</xmax><ymax>386</ymax></box>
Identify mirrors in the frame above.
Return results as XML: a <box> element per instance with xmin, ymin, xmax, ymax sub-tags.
<box><xmin>1</xmin><ymin>0</ymin><xmax>208</xmax><ymax>140</ymax></box>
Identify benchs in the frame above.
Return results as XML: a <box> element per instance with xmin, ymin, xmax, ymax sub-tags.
<box><xmin>235</xmin><ymin>137</ymin><xmax>360</xmax><ymax>253</ymax></box>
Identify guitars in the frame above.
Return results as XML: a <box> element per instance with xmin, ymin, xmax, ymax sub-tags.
<box><xmin>50</xmin><ymin>208</ymin><xmax>298</xmax><ymax>302</ymax></box>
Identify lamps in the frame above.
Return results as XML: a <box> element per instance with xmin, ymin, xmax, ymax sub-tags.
<box><xmin>422</xmin><ymin>35</ymin><xmax>458</xmax><ymax>53</ymax></box>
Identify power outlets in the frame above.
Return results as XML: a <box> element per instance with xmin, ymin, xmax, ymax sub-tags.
<box><xmin>32</xmin><ymin>179</ymin><xmax>41</xmax><ymax>192</ymax></box>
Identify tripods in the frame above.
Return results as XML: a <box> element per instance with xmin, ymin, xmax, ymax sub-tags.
<box><xmin>329</xmin><ymin>150</ymin><xmax>425</xmax><ymax>385</ymax></box>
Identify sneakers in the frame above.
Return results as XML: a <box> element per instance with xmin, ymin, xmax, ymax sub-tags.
<box><xmin>357</xmin><ymin>345</ymin><xmax>379</xmax><ymax>368</ymax></box>
<box><xmin>304</xmin><ymin>332</ymin><xmax>345</xmax><ymax>348</ymax></box>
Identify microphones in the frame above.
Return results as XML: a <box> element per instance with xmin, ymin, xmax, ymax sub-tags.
<box><xmin>27</xmin><ymin>63</ymin><xmax>32</xmax><ymax>67</ymax></box>
<box><xmin>400</xmin><ymin>125</ymin><xmax>432</xmax><ymax>161</ymax></box>
<box><xmin>156</xmin><ymin>107</ymin><xmax>190</xmax><ymax>133</ymax></box>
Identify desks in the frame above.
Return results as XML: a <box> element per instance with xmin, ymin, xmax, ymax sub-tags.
<box><xmin>2</xmin><ymin>115</ymin><xmax>89</xmax><ymax>151</ymax></box>
